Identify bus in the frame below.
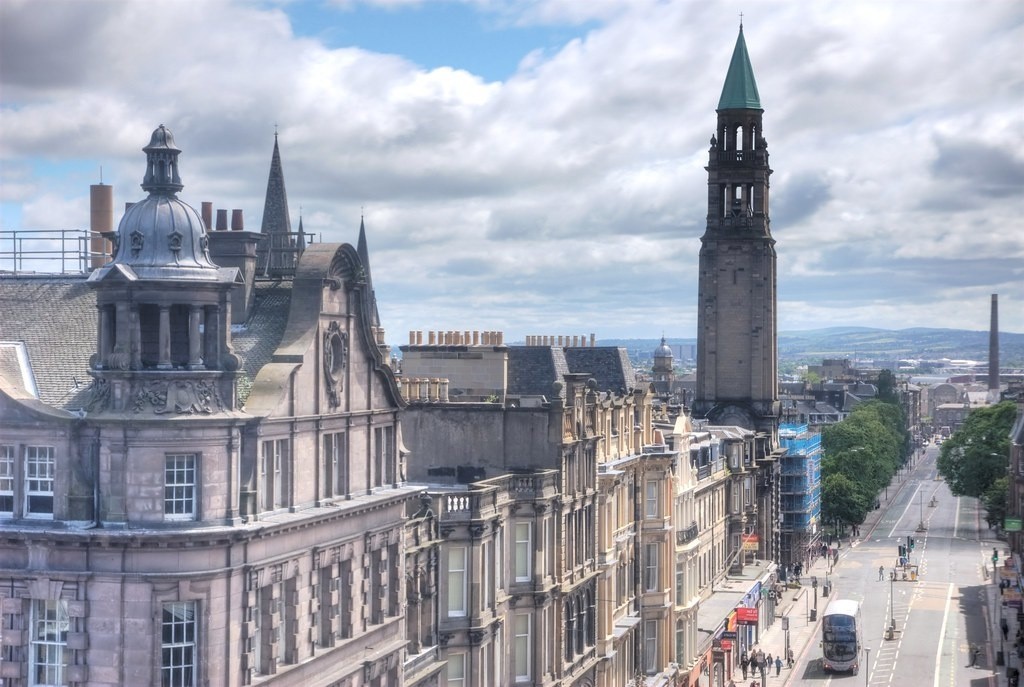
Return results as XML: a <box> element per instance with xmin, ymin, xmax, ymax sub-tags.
<box><xmin>820</xmin><ymin>599</ymin><xmax>862</xmax><ymax>676</ymax></box>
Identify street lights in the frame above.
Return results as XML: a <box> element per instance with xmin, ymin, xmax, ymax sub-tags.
<box><xmin>1007</xmin><ymin>650</ymin><xmax>1018</xmax><ymax>687</ymax></box>
<box><xmin>782</xmin><ymin>617</ymin><xmax>789</xmax><ymax>660</ymax></box>
<box><xmin>756</xmin><ymin>648</ymin><xmax>765</xmax><ymax>686</ymax></box>
<box><xmin>1000</xmin><ymin>605</ymin><xmax>1009</xmax><ymax>664</ymax></box>
<box><xmin>864</xmin><ymin>646</ymin><xmax>871</xmax><ymax>687</ymax></box>
<box><xmin>890</xmin><ymin>571</ymin><xmax>893</xmax><ymax>626</ymax></box>
<box><xmin>827</xmin><ymin>533</ymin><xmax>832</xmax><ymax>568</ymax></box>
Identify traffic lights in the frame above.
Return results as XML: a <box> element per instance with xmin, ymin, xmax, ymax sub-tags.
<box><xmin>902</xmin><ymin>546</ymin><xmax>906</xmax><ymax>557</ymax></box>
<box><xmin>910</xmin><ymin>538</ymin><xmax>914</xmax><ymax>548</ymax></box>
<box><xmin>993</xmin><ymin>548</ymin><xmax>999</xmax><ymax>561</ymax></box>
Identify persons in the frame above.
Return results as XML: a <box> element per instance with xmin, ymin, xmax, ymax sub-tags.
<box><xmin>1007</xmin><ymin>580</ymin><xmax>1011</xmax><ymax>588</ymax></box>
<box><xmin>741</xmin><ymin>648</ymin><xmax>765</xmax><ymax>679</ymax></box>
<box><xmin>999</xmin><ymin>579</ymin><xmax>1005</xmax><ymax>595</ymax></box>
<box><xmin>852</xmin><ymin>524</ymin><xmax>859</xmax><ymax>536</ymax></box>
<box><xmin>993</xmin><ymin>547</ymin><xmax>998</xmax><ymax>557</ymax></box>
<box><xmin>794</xmin><ymin>562</ymin><xmax>804</xmax><ymax>578</ymax></box>
<box><xmin>1002</xmin><ymin>621</ymin><xmax>1009</xmax><ymax>640</ymax></box>
<box><xmin>1016</xmin><ymin>630</ymin><xmax>1020</xmax><ymax>638</ymax></box>
<box><xmin>822</xmin><ymin>543</ymin><xmax>827</xmax><ymax>558</ymax></box>
<box><xmin>775</xmin><ymin>656</ymin><xmax>783</xmax><ymax>676</ymax></box>
<box><xmin>728</xmin><ymin>680</ymin><xmax>736</xmax><ymax>687</ymax></box>
<box><xmin>970</xmin><ymin>642</ymin><xmax>981</xmax><ymax>668</ymax></box>
<box><xmin>765</xmin><ymin>653</ymin><xmax>773</xmax><ymax>669</ymax></box>
<box><xmin>750</xmin><ymin>680</ymin><xmax>760</xmax><ymax>687</ymax></box>
<box><xmin>878</xmin><ymin>566</ymin><xmax>885</xmax><ymax>581</ymax></box>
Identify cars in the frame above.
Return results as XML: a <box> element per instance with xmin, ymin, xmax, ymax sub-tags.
<box><xmin>923</xmin><ymin>440</ymin><xmax>929</xmax><ymax>446</ymax></box>
<box><xmin>934</xmin><ymin>437</ymin><xmax>940</xmax><ymax>443</ymax></box>
<box><xmin>936</xmin><ymin>439</ymin><xmax>942</xmax><ymax>445</ymax></box>
